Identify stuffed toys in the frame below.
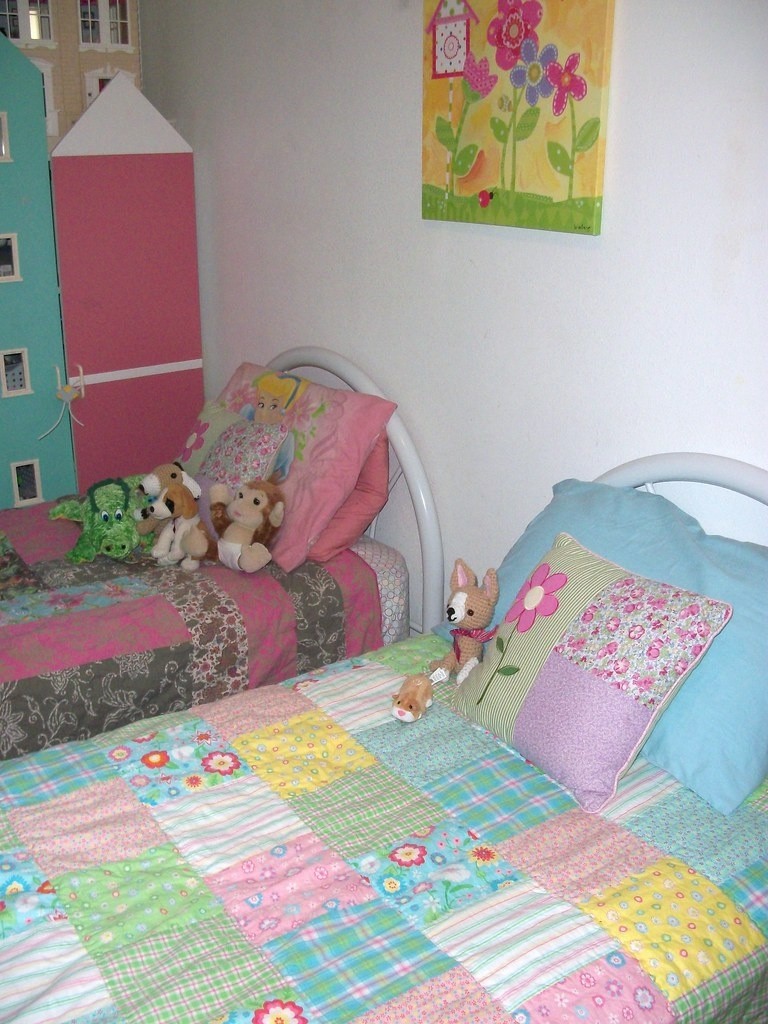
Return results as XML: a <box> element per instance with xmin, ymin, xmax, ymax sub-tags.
<box><xmin>49</xmin><ymin>472</ymin><xmax>156</xmax><ymax>566</ymax></box>
<box><xmin>429</xmin><ymin>557</ymin><xmax>499</xmax><ymax>684</ymax></box>
<box><xmin>182</xmin><ymin>481</ymin><xmax>285</xmax><ymax>574</ymax></box>
<box><xmin>392</xmin><ymin>674</ymin><xmax>434</xmax><ymax>722</ymax></box>
<box><xmin>149</xmin><ymin>484</ymin><xmax>210</xmax><ymax>572</ymax></box>
<box><xmin>138</xmin><ymin>461</ymin><xmax>201</xmax><ymax>499</ymax></box>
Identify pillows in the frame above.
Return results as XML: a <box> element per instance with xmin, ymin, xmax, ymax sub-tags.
<box><xmin>431</xmin><ymin>479</ymin><xmax>768</xmax><ymax>814</ymax></box>
<box><xmin>170</xmin><ymin>362</ymin><xmax>398</xmax><ymax>573</ymax></box>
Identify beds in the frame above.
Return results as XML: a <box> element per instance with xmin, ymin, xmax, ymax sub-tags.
<box><xmin>0</xmin><ymin>451</ymin><xmax>768</xmax><ymax>1024</ymax></box>
<box><xmin>0</xmin><ymin>348</ymin><xmax>450</xmax><ymax>763</ymax></box>
